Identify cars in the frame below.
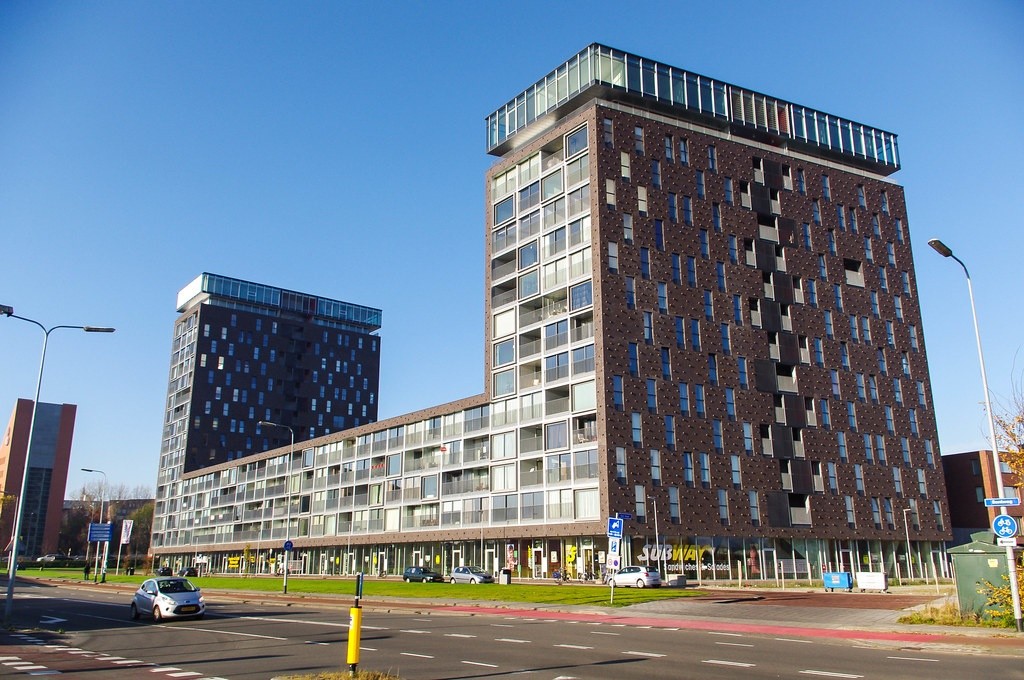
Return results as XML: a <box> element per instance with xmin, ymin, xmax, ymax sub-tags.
<box><xmin>403</xmin><ymin>566</ymin><xmax>445</xmax><ymax>583</ymax></box>
<box><xmin>16</xmin><ymin>563</ymin><xmax>25</xmax><ymax>570</ymax></box>
<box><xmin>449</xmin><ymin>566</ymin><xmax>495</xmax><ymax>584</ymax></box>
<box><xmin>130</xmin><ymin>577</ymin><xmax>206</xmax><ymax>623</ymax></box>
<box><xmin>177</xmin><ymin>566</ymin><xmax>198</xmax><ymax>577</ymax></box>
<box><xmin>607</xmin><ymin>565</ymin><xmax>661</xmax><ymax>589</ymax></box>
<box><xmin>36</xmin><ymin>553</ymin><xmax>116</xmax><ymax>562</ymax></box>
<box><xmin>155</xmin><ymin>567</ymin><xmax>173</xmax><ymax>576</ymax></box>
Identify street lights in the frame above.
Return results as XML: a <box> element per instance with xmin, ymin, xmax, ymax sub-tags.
<box><xmin>903</xmin><ymin>509</ymin><xmax>914</xmax><ymax>581</ymax></box>
<box><xmin>344</xmin><ymin>521</ymin><xmax>350</xmax><ymax>574</ymax></box>
<box><xmin>259</xmin><ymin>421</ymin><xmax>294</xmax><ymax>594</ymax></box>
<box><xmin>0</xmin><ymin>304</ymin><xmax>116</xmax><ymax>619</ymax></box>
<box><xmin>81</xmin><ymin>469</ymin><xmax>106</xmax><ymax>582</ymax></box>
<box><xmin>25</xmin><ymin>512</ymin><xmax>34</xmax><ymax>556</ymax></box>
<box><xmin>477</xmin><ymin>510</ymin><xmax>483</xmax><ymax>569</ymax></box>
<box><xmin>648</xmin><ymin>496</ymin><xmax>660</xmax><ymax>575</ymax></box>
<box><xmin>928</xmin><ymin>238</ymin><xmax>1023</xmax><ymax>632</ymax></box>
<box><xmin>194</xmin><ymin>536</ymin><xmax>199</xmax><ymax>568</ymax></box>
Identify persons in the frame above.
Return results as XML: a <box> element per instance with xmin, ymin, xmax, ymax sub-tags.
<box><xmin>508</xmin><ymin>545</ymin><xmax>515</xmax><ymax>569</ymax></box>
<box><xmin>84</xmin><ymin>563</ymin><xmax>90</xmax><ymax>580</ymax></box>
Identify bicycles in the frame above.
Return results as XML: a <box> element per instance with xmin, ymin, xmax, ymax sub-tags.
<box><xmin>552</xmin><ymin>567</ymin><xmax>572</xmax><ymax>583</ymax></box>
